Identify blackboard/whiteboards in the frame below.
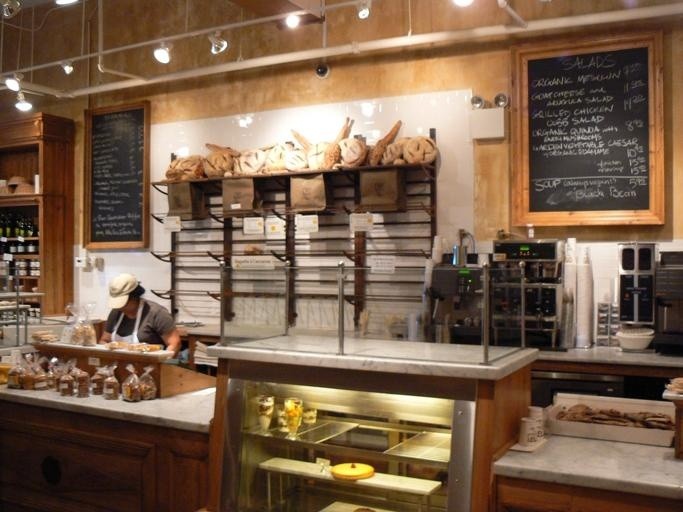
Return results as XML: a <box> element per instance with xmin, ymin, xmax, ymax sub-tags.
<box><xmin>82</xmin><ymin>100</ymin><xmax>150</xmax><ymax>250</ymax></box>
<box><xmin>511</xmin><ymin>27</ymin><xmax>666</xmax><ymax>227</ymax></box>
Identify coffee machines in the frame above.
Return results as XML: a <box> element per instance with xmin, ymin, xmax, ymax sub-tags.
<box><xmin>650</xmin><ymin>250</ymin><xmax>683</xmax><ymax>353</ymax></box>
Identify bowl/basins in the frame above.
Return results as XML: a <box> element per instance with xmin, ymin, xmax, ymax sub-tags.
<box><xmin>616</xmin><ymin>329</ymin><xmax>652</xmax><ymax>351</ymax></box>
<box><xmin>0</xmin><ymin>175</ymin><xmax>34</xmax><ymax>196</ymax></box>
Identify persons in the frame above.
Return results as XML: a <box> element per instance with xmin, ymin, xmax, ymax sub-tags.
<box><xmin>94</xmin><ymin>274</ymin><xmax>182</xmax><ymax>362</ymax></box>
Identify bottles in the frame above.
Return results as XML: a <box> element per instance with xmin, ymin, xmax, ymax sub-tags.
<box><xmin>457</xmin><ymin>271</ymin><xmax>474</xmax><ymax>293</ymax></box>
<box><xmin>525</xmin><ymin>225</ymin><xmax>535</xmax><ymax>239</ymax></box>
<box><xmin>0</xmin><ymin>209</ymin><xmax>39</xmax><ymax>255</ymax></box>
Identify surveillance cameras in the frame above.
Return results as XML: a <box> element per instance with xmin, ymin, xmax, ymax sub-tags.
<box><xmin>316</xmin><ymin>65</ymin><xmax>329</xmax><ymax>78</ymax></box>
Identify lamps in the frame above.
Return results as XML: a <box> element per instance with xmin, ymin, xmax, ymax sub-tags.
<box><xmin>466</xmin><ymin>91</ymin><xmax>511</xmax><ymax>143</ymax></box>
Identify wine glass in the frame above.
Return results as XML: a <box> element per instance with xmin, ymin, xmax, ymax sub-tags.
<box><xmin>256</xmin><ymin>397</ymin><xmax>274</xmax><ymax>437</ymax></box>
<box><xmin>284</xmin><ymin>397</ymin><xmax>303</xmax><ymax>440</ymax></box>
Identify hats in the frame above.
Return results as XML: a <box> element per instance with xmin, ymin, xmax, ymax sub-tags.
<box><xmin>106</xmin><ymin>272</ymin><xmax>139</xmax><ymax>310</ymax></box>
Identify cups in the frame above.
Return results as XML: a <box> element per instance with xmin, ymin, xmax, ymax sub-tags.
<box><xmin>527</xmin><ymin>406</ymin><xmax>544</xmax><ymax>442</ymax></box>
<box><xmin>435</xmin><ymin>323</ymin><xmax>450</xmax><ymax>343</ymax></box>
<box><xmin>302</xmin><ymin>407</ymin><xmax>316</xmax><ymax>426</ymax></box>
<box><xmin>406</xmin><ymin>312</ymin><xmax>424</xmax><ymax>341</ymax></box>
<box><xmin>422</xmin><ymin>235</ymin><xmax>443</xmax><ymax>313</ymax></box>
<box><xmin>451</xmin><ymin>245</ymin><xmax>465</xmax><ymax>267</ymax></box>
<box><xmin>562</xmin><ymin>238</ymin><xmax>594</xmax><ymax>348</ymax></box>
<box><xmin>275</xmin><ymin>404</ymin><xmax>287</xmax><ymax>432</ymax></box>
<box><xmin>518</xmin><ymin>416</ymin><xmax>537</xmax><ymax>446</ymax></box>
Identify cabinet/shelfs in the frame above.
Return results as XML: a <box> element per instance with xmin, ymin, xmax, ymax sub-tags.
<box><xmin>204</xmin><ymin>335</ymin><xmax>540</xmax><ymax>512</ymax></box>
<box><xmin>0</xmin><ymin>108</ymin><xmax>74</xmax><ymax>323</ymax></box>
<box><xmin>149</xmin><ymin>126</ymin><xmax>443</xmax><ymax>345</ymax></box>
<box><xmin>484</xmin><ymin>252</ymin><xmax>565</xmax><ymax>351</ymax></box>
